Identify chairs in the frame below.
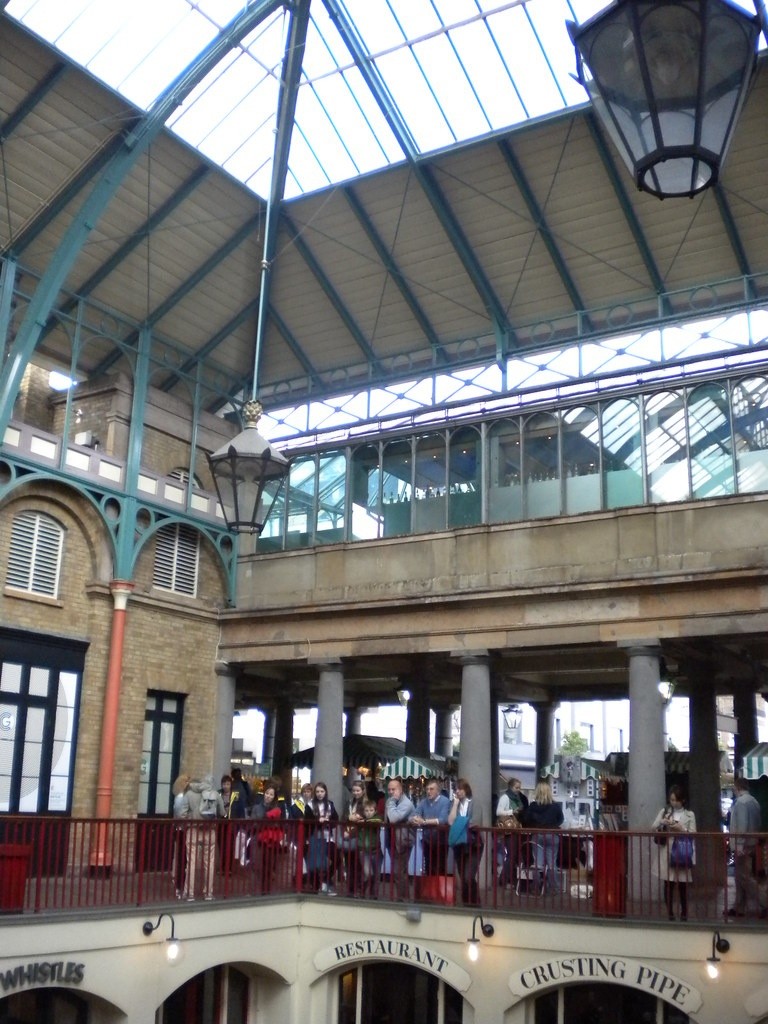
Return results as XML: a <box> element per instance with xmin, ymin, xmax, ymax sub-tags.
<box><xmin>516</xmin><ymin>841</ymin><xmax>552</xmax><ymax>898</ymax></box>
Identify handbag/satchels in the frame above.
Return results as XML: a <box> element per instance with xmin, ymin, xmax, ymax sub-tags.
<box><xmin>516</xmin><ymin>863</ymin><xmax>548</xmax><ymax>898</ymax></box>
<box><xmin>546</xmin><ymin>867</ymin><xmax>567</xmax><ymax>893</ymax></box>
<box><xmin>496</xmin><ymin>814</ymin><xmax>522</xmax><ymax>835</ymax></box>
<box><xmin>654</xmin><ymin>808</ymin><xmax>667</xmax><ymax>845</ymax></box>
<box><xmin>419</xmin><ymin>873</ymin><xmax>456</xmax><ymax>906</ymax></box>
<box><xmin>669</xmin><ymin>837</ymin><xmax>693</xmax><ymax>870</ymax></box>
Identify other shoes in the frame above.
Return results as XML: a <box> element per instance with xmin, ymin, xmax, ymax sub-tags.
<box><xmin>178</xmin><ymin>894</ymin><xmax>194</xmax><ymax>902</ymax></box>
<box><xmin>680</xmin><ymin>913</ymin><xmax>688</xmax><ymax>922</ymax></box>
<box><xmin>204</xmin><ymin>893</ymin><xmax>215</xmax><ymax>901</ymax></box>
<box><xmin>669</xmin><ymin>915</ymin><xmax>675</xmax><ymax>921</ymax></box>
<box><xmin>723</xmin><ymin>909</ymin><xmax>745</xmax><ymax>917</ymax></box>
<box><xmin>318</xmin><ymin>887</ymin><xmax>337</xmax><ymax>897</ymax></box>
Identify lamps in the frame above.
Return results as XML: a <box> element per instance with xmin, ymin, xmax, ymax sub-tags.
<box><xmin>564</xmin><ymin>0</ymin><xmax>768</xmax><ymax>202</ymax></box>
<box><xmin>502</xmin><ymin>704</ymin><xmax>524</xmax><ymax>729</ymax></box>
<box><xmin>466</xmin><ymin>915</ymin><xmax>493</xmax><ymax>961</ymax></box>
<box><xmin>204</xmin><ymin>0</ymin><xmax>313</xmax><ymax>538</ymax></box>
<box><xmin>661</xmin><ymin>678</ymin><xmax>679</xmax><ymax>705</ymax></box>
<box><xmin>708</xmin><ymin>930</ymin><xmax>730</xmax><ymax>978</ymax></box>
<box><xmin>143</xmin><ymin>913</ymin><xmax>179</xmax><ymax>959</ymax></box>
<box><xmin>395</xmin><ymin>683</ymin><xmax>408</xmax><ymax>706</ymax></box>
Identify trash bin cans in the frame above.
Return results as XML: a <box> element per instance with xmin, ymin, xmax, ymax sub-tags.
<box><xmin>0</xmin><ymin>841</ymin><xmax>30</xmax><ymax>914</ymax></box>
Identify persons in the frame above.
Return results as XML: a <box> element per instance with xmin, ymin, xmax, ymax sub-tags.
<box><xmin>171</xmin><ymin>771</ymin><xmax>565</xmax><ymax>907</ymax></box>
<box><xmin>652</xmin><ymin>785</ymin><xmax>698</xmax><ymax>921</ymax></box>
<box><xmin>726</xmin><ymin>777</ymin><xmax>762</xmax><ymax>917</ymax></box>
